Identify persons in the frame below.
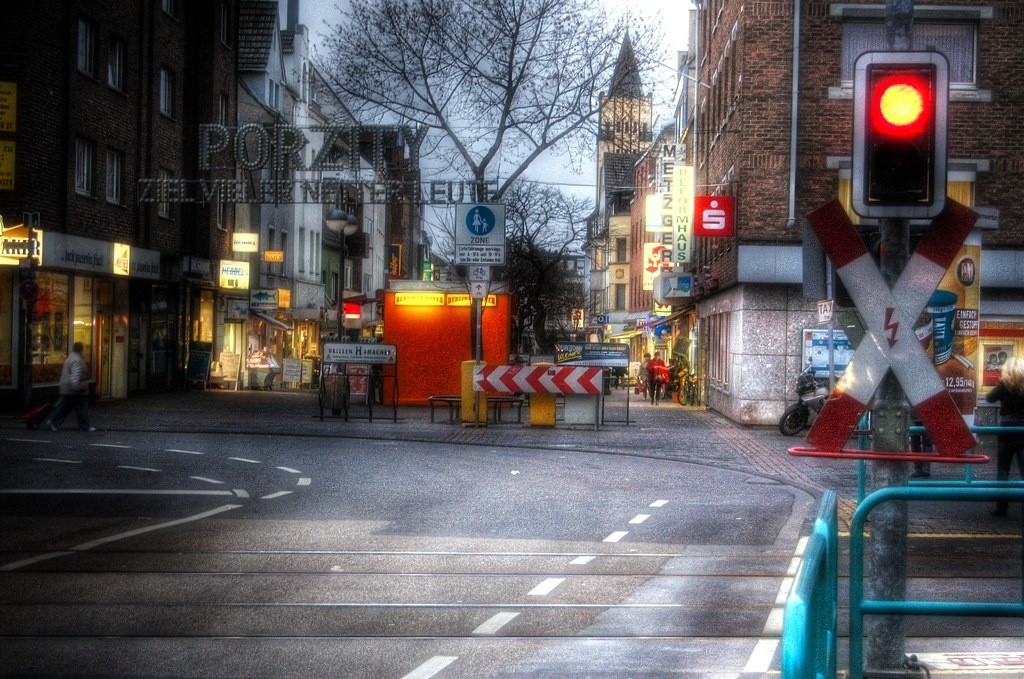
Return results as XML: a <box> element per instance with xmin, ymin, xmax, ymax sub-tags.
<box><xmin>637</xmin><ymin>352</ymin><xmax>666</xmax><ymax>405</ymax></box>
<box><xmin>986</xmin><ymin>356</ymin><xmax>1024</xmax><ymax>516</ymax></box>
<box><xmin>910</xmin><ymin>411</ymin><xmax>929</xmax><ymax>477</ymax></box>
<box><xmin>46</xmin><ymin>342</ymin><xmax>96</xmax><ymax>431</ymax></box>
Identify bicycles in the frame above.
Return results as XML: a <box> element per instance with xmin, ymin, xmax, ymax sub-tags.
<box><xmin>678</xmin><ymin>369</ymin><xmax>697</xmax><ymax>407</ymax></box>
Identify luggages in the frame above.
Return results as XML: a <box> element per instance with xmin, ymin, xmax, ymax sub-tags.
<box><xmin>18</xmin><ymin>396</ymin><xmax>61</xmax><ymax>430</ymax></box>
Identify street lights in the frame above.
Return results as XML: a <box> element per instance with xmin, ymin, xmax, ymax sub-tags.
<box><xmin>325</xmin><ymin>207</ymin><xmax>361</xmax><ymax>417</ymax></box>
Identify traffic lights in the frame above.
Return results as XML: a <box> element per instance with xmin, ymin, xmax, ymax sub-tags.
<box><xmin>849</xmin><ymin>50</ymin><xmax>950</xmax><ymax>222</ymax></box>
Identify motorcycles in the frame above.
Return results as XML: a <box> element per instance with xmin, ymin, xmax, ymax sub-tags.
<box><xmin>778</xmin><ymin>354</ymin><xmax>831</xmax><ymax>438</ymax></box>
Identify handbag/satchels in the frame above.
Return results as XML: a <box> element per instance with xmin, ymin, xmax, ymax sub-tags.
<box><xmin>654</xmin><ymin>367</ymin><xmax>670</xmax><ymax>383</ymax></box>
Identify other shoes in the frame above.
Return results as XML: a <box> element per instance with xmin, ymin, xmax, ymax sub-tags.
<box><xmin>911</xmin><ymin>468</ymin><xmax>930</xmax><ymax>478</ymax></box>
<box><xmin>45</xmin><ymin>420</ymin><xmax>58</xmax><ymax>431</ymax></box>
<box><xmin>656</xmin><ymin>399</ymin><xmax>660</xmax><ymax>405</ymax></box>
<box><xmin>989</xmin><ymin>510</ymin><xmax>1007</xmax><ymax>517</ymax></box>
<box><xmin>84</xmin><ymin>426</ymin><xmax>96</xmax><ymax>432</ymax></box>
<box><xmin>651</xmin><ymin>397</ymin><xmax>655</xmax><ymax>405</ymax></box>
